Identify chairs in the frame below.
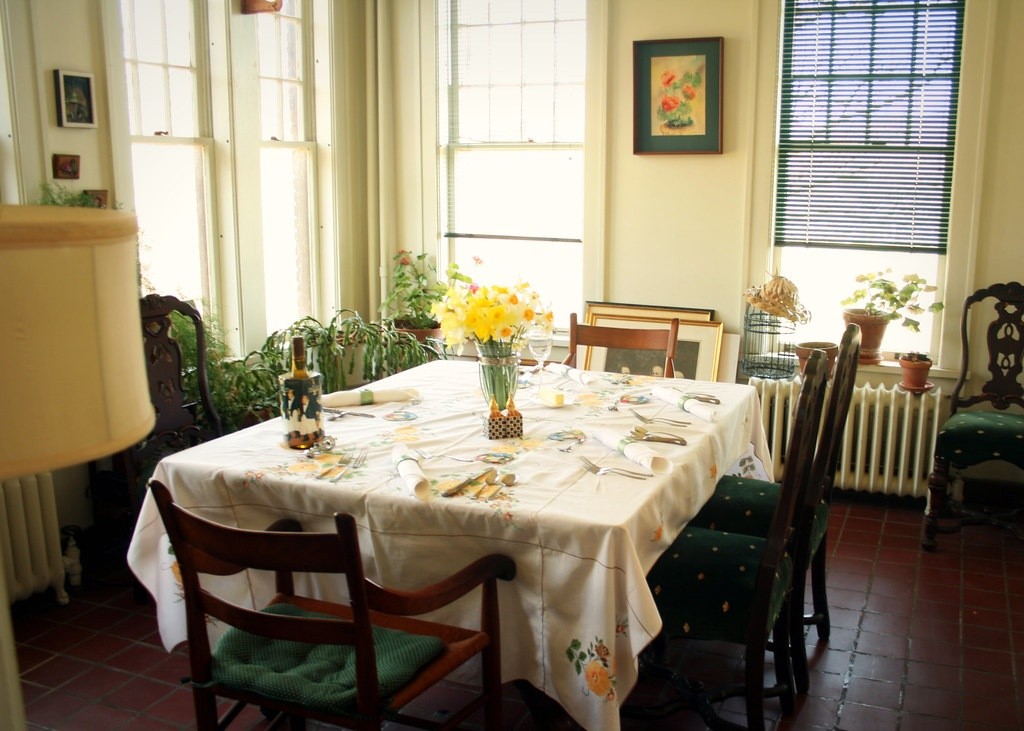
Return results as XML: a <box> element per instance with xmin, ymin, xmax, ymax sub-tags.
<box><xmin>922</xmin><ymin>282</ymin><xmax>1024</xmax><ymax>552</ymax></box>
<box><xmin>108</xmin><ymin>293</ymin><xmax>222</xmax><ymax>504</ymax></box>
<box><xmin>568</xmin><ymin>313</ymin><xmax>678</xmax><ymax>379</ymax></box>
<box><xmin>690</xmin><ymin>322</ymin><xmax>866</xmax><ymax>693</ymax></box>
<box><xmin>150</xmin><ymin>478</ymin><xmax>517</xmax><ymax>731</ymax></box>
<box><xmin>619</xmin><ymin>350</ymin><xmax>828</xmax><ymax>731</ymax></box>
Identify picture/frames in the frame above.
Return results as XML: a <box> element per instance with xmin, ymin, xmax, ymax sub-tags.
<box><xmin>56</xmin><ymin>70</ymin><xmax>98</xmax><ymax>128</ymax></box>
<box><xmin>584</xmin><ymin>301</ymin><xmax>725</xmax><ymax>386</ymax></box>
<box><xmin>632</xmin><ymin>36</ymin><xmax>724</xmax><ymax>155</ymax></box>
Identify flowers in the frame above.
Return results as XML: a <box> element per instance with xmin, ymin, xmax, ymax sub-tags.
<box><xmin>430</xmin><ymin>258</ymin><xmax>556</xmax><ymax>410</ymax></box>
<box><xmin>377</xmin><ymin>249</ymin><xmax>442</xmax><ymax>329</ymax></box>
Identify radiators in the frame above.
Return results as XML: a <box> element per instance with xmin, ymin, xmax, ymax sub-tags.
<box><xmin>0</xmin><ymin>470</ymin><xmax>77</xmax><ymax>604</ymax></box>
<box><xmin>747</xmin><ymin>375</ymin><xmax>942</xmax><ymax>514</ymax></box>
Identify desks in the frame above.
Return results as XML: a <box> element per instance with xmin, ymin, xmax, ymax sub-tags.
<box><xmin>125</xmin><ymin>358</ymin><xmax>769</xmax><ymax>731</ymax></box>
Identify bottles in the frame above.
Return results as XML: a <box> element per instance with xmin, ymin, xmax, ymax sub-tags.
<box><xmin>277</xmin><ymin>336</ymin><xmax>324</xmax><ymax>449</ymax></box>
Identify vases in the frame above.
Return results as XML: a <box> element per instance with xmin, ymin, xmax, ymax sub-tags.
<box><xmin>476</xmin><ymin>343</ymin><xmax>523</xmax><ymax>438</ymax></box>
<box><xmin>794</xmin><ymin>342</ymin><xmax>838</xmax><ymax>379</ymax></box>
<box><xmin>393</xmin><ymin>319</ymin><xmax>441</xmax><ymax>345</ymax></box>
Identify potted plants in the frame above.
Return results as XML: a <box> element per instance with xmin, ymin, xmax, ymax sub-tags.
<box><xmin>181</xmin><ymin>351</ymin><xmax>286</xmax><ymax>437</ymax></box>
<box><xmin>845</xmin><ymin>270</ymin><xmax>945</xmax><ymax>365</ymax></box>
<box><xmin>261</xmin><ymin>309</ymin><xmax>442</xmax><ymax>394</ymax></box>
<box><xmin>898</xmin><ymin>353</ymin><xmax>936</xmax><ymax>393</ymax></box>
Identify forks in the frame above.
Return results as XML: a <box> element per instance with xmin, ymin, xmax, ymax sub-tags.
<box><xmin>302</xmin><ymin>365</ymin><xmax>719</xmax><ymax>501</ymax></box>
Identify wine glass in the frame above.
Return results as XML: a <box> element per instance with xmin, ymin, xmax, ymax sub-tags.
<box><xmin>528</xmin><ymin>322</ymin><xmax>552</xmax><ymax>393</ymax></box>
